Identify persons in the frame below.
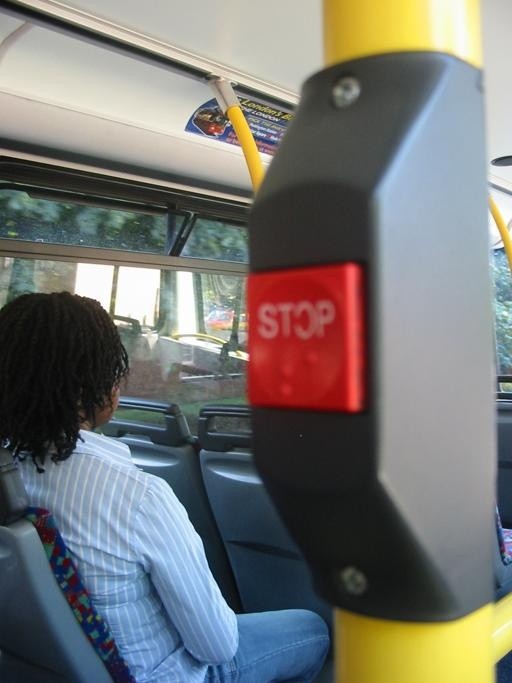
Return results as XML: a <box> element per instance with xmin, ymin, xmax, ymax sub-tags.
<box><xmin>0</xmin><ymin>288</ymin><xmax>334</xmax><ymax>681</ymax></box>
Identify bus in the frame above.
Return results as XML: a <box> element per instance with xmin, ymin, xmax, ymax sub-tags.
<box><xmin>195</xmin><ymin>106</ymin><xmax>228</xmax><ymax>137</ymax></box>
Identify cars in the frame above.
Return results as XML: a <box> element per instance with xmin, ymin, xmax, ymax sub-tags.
<box><xmin>206</xmin><ymin>304</ymin><xmax>249</xmax><ymax>331</ymax></box>
